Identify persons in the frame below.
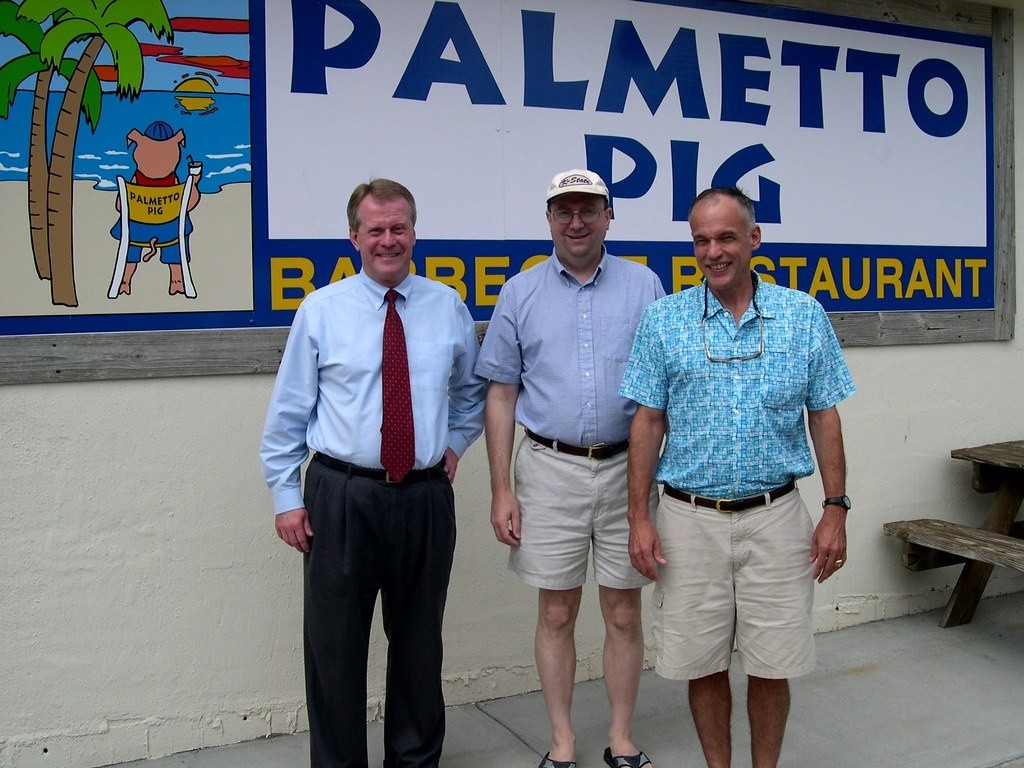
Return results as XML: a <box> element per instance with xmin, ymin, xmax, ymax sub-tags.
<box><xmin>258</xmin><ymin>178</ymin><xmax>492</xmax><ymax>768</ymax></box>
<box><xmin>616</xmin><ymin>186</ymin><xmax>860</xmax><ymax>768</ymax></box>
<box><xmin>472</xmin><ymin>165</ymin><xmax>675</xmax><ymax>768</ymax></box>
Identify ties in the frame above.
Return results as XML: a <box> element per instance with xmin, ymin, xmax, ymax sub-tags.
<box><xmin>375</xmin><ymin>290</ymin><xmax>418</xmax><ymax>484</ymax></box>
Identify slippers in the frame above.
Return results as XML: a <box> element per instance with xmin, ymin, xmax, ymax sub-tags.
<box><xmin>536</xmin><ymin>753</ymin><xmax>575</xmax><ymax>767</ymax></box>
<box><xmin>604</xmin><ymin>747</ymin><xmax>655</xmax><ymax>768</ymax></box>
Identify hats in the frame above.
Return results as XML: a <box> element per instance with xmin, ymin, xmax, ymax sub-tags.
<box><xmin>544</xmin><ymin>166</ymin><xmax>614</xmax><ymax>203</ymax></box>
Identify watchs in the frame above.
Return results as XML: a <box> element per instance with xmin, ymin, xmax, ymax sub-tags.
<box><xmin>820</xmin><ymin>495</ymin><xmax>852</xmax><ymax>511</ymax></box>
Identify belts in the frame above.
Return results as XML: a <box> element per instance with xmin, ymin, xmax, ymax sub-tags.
<box><xmin>659</xmin><ymin>480</ymin><xmax>802</xmax><ymax>514</ymax></box>
<box><xmin>309</xmin><ymin>448</ymin><xmax>455</xmax><ymax>484</ymax></box>
<box><xmin>521</xmin><ymin>425</ymin><xmax>633</xmax><ymax>463</ymax></box>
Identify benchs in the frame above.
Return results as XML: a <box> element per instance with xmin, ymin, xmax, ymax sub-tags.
<box><xmin>885</xmin><ymin>518</ymin><xmax>1023</xmax><ymax>628</ymax></box>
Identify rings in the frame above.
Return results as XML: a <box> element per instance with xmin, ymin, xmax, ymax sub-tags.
<box><xmin>833</xmin><ymin>559</ymin><xmax>843</xmax><ymax>569</ymax></box>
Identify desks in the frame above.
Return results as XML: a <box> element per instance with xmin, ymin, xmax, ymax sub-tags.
<box><xmin>950</xmin><ymin>440</ymin><xmax>1023</xmax><ymax>535</ymax></box>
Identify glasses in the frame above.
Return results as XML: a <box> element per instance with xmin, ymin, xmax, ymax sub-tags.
<box><xmin>700</xmin><ymin>314</ymin><xmax>766</xmax><ymax>364</ymax></box>
<box><xmin>547</xmin><ymin>206</ymin><xmax>611</xmax><ymax>226</ymax></box>
